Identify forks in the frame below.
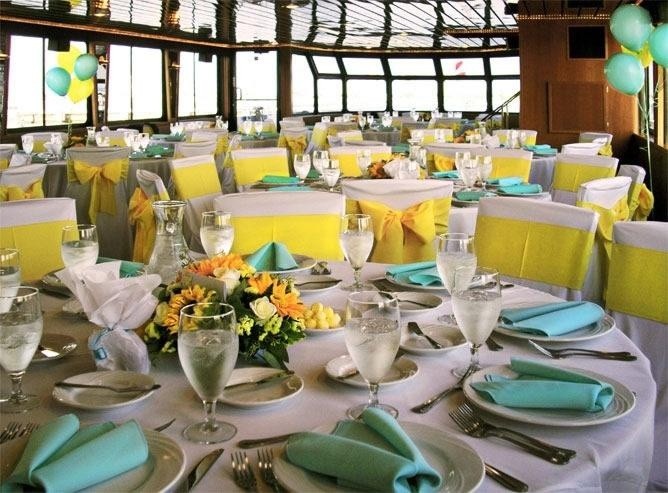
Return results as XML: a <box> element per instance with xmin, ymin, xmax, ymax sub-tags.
<box><xmin>0</xmin><ymin>420</ymin><xmax>23</xmax><ymax>445</ymax></box>
<box><xmin>230</xmin><ymin>450</ymin><xmax>259</xmax><ymax>493</ymax></box>
<box><xmin>529</xmin><ymin>339</ymin><xmax>638</xmax><ymax>362</ymax></box>
<box><xmin>456</xmin><ymin>401</ymin><xmax>577</xmax><ymax>459</ymax></box>
<box><xmin>448</xmin><ymin>408</ymin><xmax>569</xmax><ymax>465</ymax></box>
<box><xmin>410</xmin><ymin>363</ymin><xmax>480</xmax><ymax>414</ymax></box>
<box><xmin>18</xmin><ymin>420</ymin><xmax>39</xmax><ymax>438</ymax></box>
<box><xmin>256</xmin><ymin>446</ymin><xmax>284</xmax><ymax>492</ymax></box>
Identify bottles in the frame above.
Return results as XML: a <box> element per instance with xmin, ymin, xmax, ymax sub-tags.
<box><xmin>86</xmin><ymin>126</ymin><xmax>97</xmax><ymax>148</ymax></box>
<box><xmin>144</xmin><ymin>199</ymin><xmax>197</xmax><ymax>284</ymax></box>
<box><xmin>406</xmin><ymin>137</ymin><xmax>424</xmax><ymax>165</ymax></box>
<box><xmin>215</xmin><ymin>114</ymin><xmax>223</xmax><ymax>128</ymax></box>
<box><xmin>357</xmin><ymin>111</ymin><xmax>363</xmax><ymax>124</ymax></box>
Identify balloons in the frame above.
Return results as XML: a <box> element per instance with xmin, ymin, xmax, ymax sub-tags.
<box><xmin>74</xmin><ymin>53</ymin><xmax>99</xmax><ymax>81</ymax></box>
<box><xmin>66</xmin><ymin>76</ymin><xmax>94</xmax><ymax>104</ymax></box>
<box><xmin>45</xmin><ymin>67</ymin><xmax>71</xmax><ymax>97</ymax></box>
<box><xmin>609</xmin><ymin>4</ymin><xmax>653</xmax><ymax>54</ymax></box>
<box><xmin>648</xmin><ymin>23</ymin><xmax>668</xmax><ymax>70</ymax></box>
<box><xmin>604</xmin><ymin>52</ymin><xmax>645</xmax><ymax>97</ymax></box>
<box><xmin>56</xmin><ymin>45</ymin><xmax>81</xmax><ymax>75</ymax></box>
<box><xmin>620</xmin><ymin>42</ymin><xmax>653</xmax><ymax>68</ymax></box>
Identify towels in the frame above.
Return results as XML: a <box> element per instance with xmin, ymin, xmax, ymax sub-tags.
<box><xmin>0</xmin><ymin>413</ymin><xmax>156</xmax><ymax>493</ymax></box>
<box><xmin>470</xmin><ymin>357</ymin><xmax>615</xmax><ymax>413</ymax></box>
<box><xmin>284</xmin><ymin>407</ymin><xmax>443</xmax><ymax>493</ymax></box>
<box><xmin>497</xmin><ymin>301</ymin><xmax>605</xmax><ymax>337</ymax></box>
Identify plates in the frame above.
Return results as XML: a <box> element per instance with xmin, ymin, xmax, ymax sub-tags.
<box><xmin>462</xmin><ymin>363</ymin><xmax>636</xmax><ymax>428</ymax></box>
<box><xmin>271</xmin><ymin>418</ymin><xmax>486</xmax><ymax>493</ymax></box>
<box><xmin>492</xmin><ymin>302</ymin><xmax>617</xmax><ymax>343</ymax></box>
<box><xmin>391</xmin><ymin>291</ymin><xmax>444</xmax><ymax>315</ymax></box>
<box><xmin>451</xmin><ymin>191</ymin><xmax>497</xmax><ymax>204</ymax></box>
<box><xmin>452</xmin><ymin>183</ymin><xmax>467</xmax><ymax>189</ymax></box>
<box><xmin>240</xmin><ymin>253</ymin><xmax>318</xmax><ymax>275</ymax></box>
<box><xmin>301</xmin><ymin>306</ymin><xmax>346</xmax><ymax>336</ymax></box>
<box><xmin>305</xmin><ymin>175</ymin><xmax>323</xmax><ymax>181</ymax></box>
<box><xmin>532</xmin><ymin>155</ymin><xmax>543</xmax><ymax>159</ymax></box>
<box><xmin>496</xmin><ymin>189</ymin><xmax>550</xmax><ymax>197</ymax></box>
<box><xmin>324</xmin><ymin>354</ymin><xmax>420</xmax><ymax>388</ymax></box>
<box><xmin>385</xmin><ymin>265</ymin><xmax>500</xmax><ymax>290</ymax></box>
<box><xmin>435</xmin><ymin>176</ymin><xmax>463</xmax><ymax>182</ymax></box>
<box><xmin>52</xmin><ymin>369</ymin><xmax>156</xmax><ymax>412</ymax></box>
<box><xmin>397</xmin><ymin>322</ymin><xmax>469</xmax><ymax>356</ymax></box>
<box><xmin>251</xmin><ymin>180</ymin><xmax>305</xmax><ymax>187</ymax></box>
<box><xmin>296</xmin><ymin>273</ymin><xmax>343</xmax><ymax>293</ymax></box>
<box><xmin>218</xmin><ymin>367</ymin><xmax>305</xmax><ymax>409</ymax></box>
<box><xmin>30</xmin><ymin>332</ymin><xmax>79</xmax><ymax>363</ymax></box>
<box><xmin>1</xmin><ymin>420</ymin><xmax>187</xmax><ymax>493</ymax></box>
<box><xmin>486</xmin><ymin>183</ymin><xmax>500</xmax><ymax>188</ymax></box>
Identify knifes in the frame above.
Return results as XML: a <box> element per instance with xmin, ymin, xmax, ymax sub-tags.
<box><xmin>20</xmin><ymin>284</ymin><xmax>70</xmax><ymax>300</ymax></box>
<box><xmin>155</xmin><ymin>417</ymin><xmax>176</xmax><ymax>433</ymax></box>
<box><xmin>174</xmin><ymin>448</ymin><xmax>225</xmax><ymax>493</ymax></box>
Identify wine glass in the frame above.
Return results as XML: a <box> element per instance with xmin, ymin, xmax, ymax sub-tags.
<box><xmin>176</xmin><ymin>302</ymin><xmax>240</xmax><ymax>445</ymax></box>
<box><xmin>322</xmin><ymin>158</ymin><xmax>341</xmax><ymax>193</ymax></box>
<box><xmin>21</xmin><ymin>135</ymin><xmax>34</xmax><ymax>156</ymax></box>
<box><xmin>430</xmin><ymin>109</ymin><xmax>463</xmax><ymax>121</ymax></box>
<box><xmin>0</xmin><ymin>248</ymin><xmax>21</xmax><ymax>316</ymax></box>
<box><xmin>218</xmin><ymin>120</ymin><xmax>229</xmax><ymax>129</ymax></box>
<box><xmin>460</xmin><ymin>159</ymin><xmax>479</xmax><ymax>192</ymax></box>
<box><xmin>416</xmin><ymin>149</ymin><xmax>427</xmax><ymax>169</ymax></box>
<box><xmin>435</xmin><ymin>232</ymin><xmax>478</xmax><ymax>326</ymax></box>
<box><xmin>507</xmin><ymin>130</ymin><xmax>519</xmax><ymax>149</ymax></box>
<box><xmin>243</xmin><ymin>120</ymin><xmax>252</xmax><ymax>136</ymax></box>
<box><xmin>469</xmin><ymin>136</ymin><xmax>482</xmax><ymax>145</ymax></box>
<box><xmin>334</xmin><ymin>113</ymin><xmax>353</xmax><ymax>122</ymax></box>
<box><xmin>359</xmin><ymin>116</ymin><xmax>367</xmax><ymax>132</ymax></box>
<box><xmin>50</xmin><ymin>133</ymin><xmax>63</xmax><ymax>155</ymax></box>
<box><xmin>123</xmin><ymin>131</ymin><xmax>149</xmax><ymax>154</ymax></box>
<box><xmin>411</xmin><ymin>128</ymin><xmax>453</xmax><ymax>143</ymax></box>
<box><xmin>320</xmin><ymin>115</ymin><xmax>330</xmax><ymax>124</ymax></box>
<box><xmin>455</xmin><ymin>152</ymin><xmax>471</xmax><ymax>176</ymax></box>
<box><xmin>367</xmin><ymin>115</ymin><xmax>374</xmax><ymax>127</ymax></box>
<box><xmin>339</xmin><ymin>213</ymin><xmax>376</xmax><ymax>293</ymax></box>
<box><xmin>293</xmin><ymin>154</ymin><xmax>312</xmax><ymax>185</ymax></box>
<box><xmin>254</xmin><ymin>120</ymin><xmax>263</xmax><ymax>136</ymax></box>
<box><xmin>312</xmin><ymin>150</ymin><xmax>330</xmax><ymax>183</ymax></box>
<box><xmin>0</xmin><ymin>285</ymin><xmax>44</xmax><ymax>415</ymax></box>
<box><xmin>343</xmin><ymin>289</ymin><xmax>401</xmax><ymax>424</ymax></box>
<box><xmin>381</xmin><ymin>111</ymin><xmax>392</xmax><ymax>128</ymax></box>
<box><xmin>168</xmin><ymin>120</ymin><xmax>211</xmax><ymax>136</ymax></box>
<box><xmin>476</xmin><ymin>155</ymin><xmax>492</xmax><ymax>192</ymax></box>
<box><xmin>60</xmin><ymin>223</ymin><xmax>99</xmax><ymax>314</ymax></box>
<box><xmin>200</xmin><ymin>210</ymin><xmax>235</xmax><ymax>260</ymax></box>
<box><xmin>517</xmin><ymin>130</ymin><xmax>527</xmax><ymax>148</ymax></box>
<box><xmin>356</xmin><ymin>148</ymin><xmax>371</xmax><ymax>179</ymax></box>
<box><xmin>410</xmin><ymin>108</ymin><xmax>417</xmax><ymax>119</ymax></box>
<box><xmin>475</xmin><ymin>127</ymin><xmax>487</xmax><ymax>140</ymax></box>
<box><xmin>450</xmin><ymin>265</ymin><xmax>504</xmax><ymax>379</ymax></box>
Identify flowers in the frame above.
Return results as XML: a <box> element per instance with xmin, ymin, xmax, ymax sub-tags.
<box><xmin>142</xmin><ymin>254</ymin><xmax>309</xmax><ymax>379</ymax></box>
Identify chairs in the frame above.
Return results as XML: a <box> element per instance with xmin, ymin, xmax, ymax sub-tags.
<box><xmin>605</xmin><ymin>222</ymin><xmax>668</xmax><ymax>489</ymax></box>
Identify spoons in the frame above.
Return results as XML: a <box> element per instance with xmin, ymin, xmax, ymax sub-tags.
<box><xmin>223</xmin><ymin>370</ymin><xmax>294</xmax><ymax>391</ymax></box>
<box><xmin>382</xmin><ymin>293</ymin><xmax>434</xmax><ymax>308</ymax></box>
<box><xmin>410</xmin><ymin>324</ymin><xmax>441</xmax><ymax>348</ymax></box>
<box><xmin>56</xmin><ymin>380</ymin><xmax>161</xmax><ymax>394</ymax></box>
